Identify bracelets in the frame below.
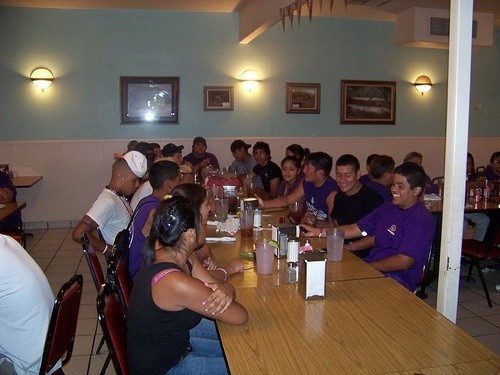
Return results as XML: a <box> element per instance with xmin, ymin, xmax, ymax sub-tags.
<box><xmin>103</xmin><ymin>244</ymin><xmax>108</xmax><ymax>254</ymax></box>
<box><xmin>217</xmin><ymin>267</ymin><xmax>227</xmax><ymax>281</ymax></box>
<box><xmin>349</xmin><ymin>242</ymin><xmax>353</xmax><ymax>251</ymax></box>
<box><xmin>318</xmin><ymin>227</ymin><xmax>323</xmax><ymax>238</ymax></box>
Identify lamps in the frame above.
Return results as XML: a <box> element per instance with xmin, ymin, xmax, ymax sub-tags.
<box><xmin>30</xmin><ymin>66</ymin><xmax>55</xmax><ymax>92</ymax></box>
<box><xmin>414</xmin><ymin>75</ymin><xmax>433</xmax><ymax>96</ymax></box>
<box><xmin>238</xmin><ymin>69</ymin><xmax>264</xmax><ymax>95</ymax></box>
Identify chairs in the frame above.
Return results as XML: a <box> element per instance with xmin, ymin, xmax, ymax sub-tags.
<box><xmin>0</xmin><ymin>227</ymin><xmax>26</xmax><ymax>249</ymax></box>
<box><xmin>37</xmin><ymin>227</ymin><xmax>133</xmax><ymax>375</ymax></box>
<box><xmin>430</xmin><ymin>167</ymin><xmax>500</xmax><ymax>307</ymax></box>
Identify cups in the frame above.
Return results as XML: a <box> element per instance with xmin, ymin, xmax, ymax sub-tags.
<box><xmin>326</xmin><ymin>228</ymin><xmax>345</xmax><ymax>261</ymax></box>
<box><xmin>234</xmin><ymin>170</ymin><xmax>238</xmax><ymax>178</ymax></box>
<box><xmin>215</xmin><ymin>198</ymin><xmax>229</xmax><ymax>223</ymax></box>
<box><xmin>289</xmin><ymin>201</ymin><xmax>303</xmax><ymax>225</ymax></box>
<box><xmin>239</xmin><ymin>209</ymin><xmax>254</xmax><ymax>236</ymax></box>
<box><xmin>253</xmin><ymin>210</ymin><xmax>262</xmax><ymax>227</ymax></box>
<box><xmin>220</xmin><ymin>166</ymin><xmax>227</xmax><ymax>175</ymax></box>
<box><xmin>247</xmin><ymin>182</ymin><xmax>256</xmax><ymax>198</ymax></box>
<box><xmin>255</xmin><ymin>239</ymin><xmax>275</xmax><ymax>274</ymax></box>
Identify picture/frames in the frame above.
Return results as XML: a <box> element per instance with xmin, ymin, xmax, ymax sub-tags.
<box><xmin>119</xmin><ymin>75</ymin><xmax>181</xmax><ymax>126</ymax></box>
<box><xmin>284</xmin><ymin>82</ymin><xmax>322</xmax><ymax>115</ymax></box>
<box><xmin>203</xmin><ymin>86</ymin><xmax>235</xmax><ymax>111</ymax></box>
<box><xmin>339</xmin><ymin>79</ymin><xmax>396</xmax><ymax>126</ymax></box>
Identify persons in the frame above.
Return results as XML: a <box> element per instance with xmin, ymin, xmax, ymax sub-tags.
<box><xmin>161</xmin><ymin>143</ymin><xmax>184</xmax><ymax>164</ymax></box>
<box><xmin>182</xmin><ymin>137</ymin><xmax>221</xmax><ymax>182</ymax></box>
<box><xmin>227</xmin><ymin>139</ymin><xmax>257</xmax><ymax>179</ymax></box>
<box><xmin>127</xmin><ymin>195</ymin><xmax>248</xmax><ymax>375</ymax></box>
<box><xmin>0</xmin><ymin>234</ymin><xmax>65</xmax><ymax>375</ymax></box>
<box><xmin>73</xmin><ymin>140</ymin><xmax>161</xmax><ymax>256</ymax></box>
<box><xmin>251</xmin><ymin>142</ymin><xmax>284</xmax><ymax>198</ymax></box>
<box><xmin>127</xmin><ymin>160</ymin><xmax>182</xmax><ymax>282</ymax></box>
<box><xmin>276</xmin><ymin>156</ymin><xmax>304</xmax><ymax>211</ymax></box>
<box><xmin>0</xmin><ymin>171</ymin><xmax>22</xmax><ymax>233</ymax></box>
<box><xmin>130</xmin><ymin>183</ymin><xmax>244</xmax><ymax>283</ymax></box>
<box><xmin>483</xmin><ymin>151</ymin><xmax>500</xmax><ymax>204</ymax></box>
<box><xmin>360</xmin><ymin>154</ymin><xmax>379</xmax><ymax>187</ymax></box>
<box><xmin>329</xmin><ymin>154</ymin><xmax>385</xmax><ymax>259</ymax></box>
<box><xmin>463</xmin><ymin>153</ymin><xmax>490</xmax><ymax>241</ymax></box>
<box><xmin>298</xmin><ymin>151</ymin><xmax>437</xmax><ymax>295</ymax></box>
<box><xmin>254</xmin><ymin>152</ymin><xmax>340</xmax><ymax>225</ymax></box>
<box><xmin>285</xmin><ymin>144</ymin><xmax>310</xmax><ymax>162</ymax></box>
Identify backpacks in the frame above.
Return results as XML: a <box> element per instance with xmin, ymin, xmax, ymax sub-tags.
<box><xmin>106</xmin><ymin>197</ymin><xmax>157</xmax><ymax>295</ymax></box>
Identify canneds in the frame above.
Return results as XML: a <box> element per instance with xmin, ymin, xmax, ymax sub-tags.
<box><xmin>473</xmin><ymin>187</ymin><xmax>482</xmax><ymax>204</ymax></box>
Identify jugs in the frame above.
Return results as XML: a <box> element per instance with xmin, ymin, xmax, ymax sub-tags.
<box><xmin>222</xmin><ymin>185</ymin><xmax>238</xmax><ymax>215</ymax></box>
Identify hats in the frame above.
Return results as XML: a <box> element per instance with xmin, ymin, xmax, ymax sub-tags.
<box><xmin>161</xmin><ymin>143</ymin><xmax>184</xmax><ymax>154</ymax></box>
<box><xmin>114</xmin><ymin>151</ymin><xmax>147</xmax><ymax>177</ymax></box>
<box><xmin>230</xmin><ymin>139</ymin><xmax>251</xmax><ymax>152</ymax></box>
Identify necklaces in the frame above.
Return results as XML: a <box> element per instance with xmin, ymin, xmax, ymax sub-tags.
<box><xmin>106</xmin><ymin>184</ymin><xmax>134</xmax><ymax>216</ymax></box>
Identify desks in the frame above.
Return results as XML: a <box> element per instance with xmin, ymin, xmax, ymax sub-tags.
<box><xmin>204</xmin><ymin>193</ymin><xmax>500</xmax><ymax>375</ymax></box>
<box><xmin>10</xmin><ymin>175</ymin><xmax>44</xmax><ymax>189</ymax></box>
<box><xmin>0</xmin><ymin>201</ymin><xmax>26</xmax><ymax>225</ymax></box>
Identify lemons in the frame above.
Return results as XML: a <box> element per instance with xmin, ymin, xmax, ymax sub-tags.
<box><xmin>268</xmin><ymin>240</ymin><xmax>280</xmax><ymax>249</ymax></box>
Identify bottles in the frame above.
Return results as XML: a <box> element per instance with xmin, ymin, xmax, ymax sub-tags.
<box><xmin>437</xmin><ymin>178</ymin><xmax>443</xmax><ymax>197</ymax></box>
<box><xmin>473</xmin><ymin>188</ymin><xmax>480</xmax><ymax>203</ymax></box>
<box><xmin>483</xmin><ymin>180</ymin><xmax>491</xmax><ymax>200</ymax></box>
<box><xmin>286</xmin><ymin>236</ymin><xmax>300</xmax><ymax>263</ymax></box>
<box><xmin>304</xmin><ymin>239</ymin><xmax>310</xmax><ymax>246</ymax></box>
<box><xmin>286</xmin><ymin>262</ymin><xmax>298</xmax><ymax>283</ymax></box>
